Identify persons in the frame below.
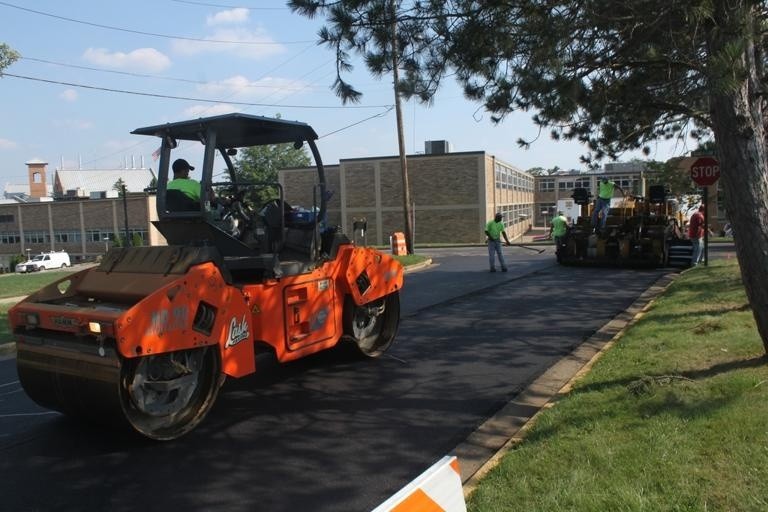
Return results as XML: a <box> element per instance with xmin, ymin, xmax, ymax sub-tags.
<box><xmin>548</xmin><ymin>210</ymin><xmax>572</xmax><ymax>257</ymax></box>
<box><xmin>687</xmin><ymin>202</ymin><xmax>714</xmax><ymax>268</ymax></box>
<box><xmin>165</xmin><ymin>158</ymin><xmax>238</xmax><ymax>235</ymax></box>
<box><xmin>483</xmin><ymin>211</ymin><xmax>510</xmax><ymax>273</ymax></box>
<box><xmin>589</xmin><ymin>174</ymin><xmax>627</xmax><ymax>235</ymax></box>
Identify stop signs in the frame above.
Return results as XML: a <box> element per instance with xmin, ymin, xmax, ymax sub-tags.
<box><xmin>691</xmin><ymin>158</ymin><xmax>720</xmax><ymax>186</ymax></box>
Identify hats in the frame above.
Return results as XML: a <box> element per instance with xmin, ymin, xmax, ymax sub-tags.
<box><xmin>172</xmin><ymin>159</ymin><xmax>195</xmax><ymax>173</ymax></box>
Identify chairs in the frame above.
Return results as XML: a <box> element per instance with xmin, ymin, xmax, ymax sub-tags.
<box><xmin>164</xmin><ymin>188</ymin><xmax>234</xmax><ymax>231</ymax></box>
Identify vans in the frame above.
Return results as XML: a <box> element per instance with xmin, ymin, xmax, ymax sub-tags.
<box><xmin>15</xmin><ymin>252</ymin><xmax>71</xmax><ymax>273</ymax></box>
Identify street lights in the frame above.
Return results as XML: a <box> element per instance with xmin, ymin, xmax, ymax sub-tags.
<box><xmin>391</xmin><ymin>32</ymin><xmax>424</xmax><ymax>255</ymax></box>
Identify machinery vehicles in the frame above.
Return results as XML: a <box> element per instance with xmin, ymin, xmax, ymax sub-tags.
<box><xmin>556</xmin><ymin>185</ymin><xmax>703</xmax><ymax>269</ymax></box>
<box><xmin>8</xmin><ymin>113</ymin><xmax>404</xmax><ymax>440</ymax></box>
